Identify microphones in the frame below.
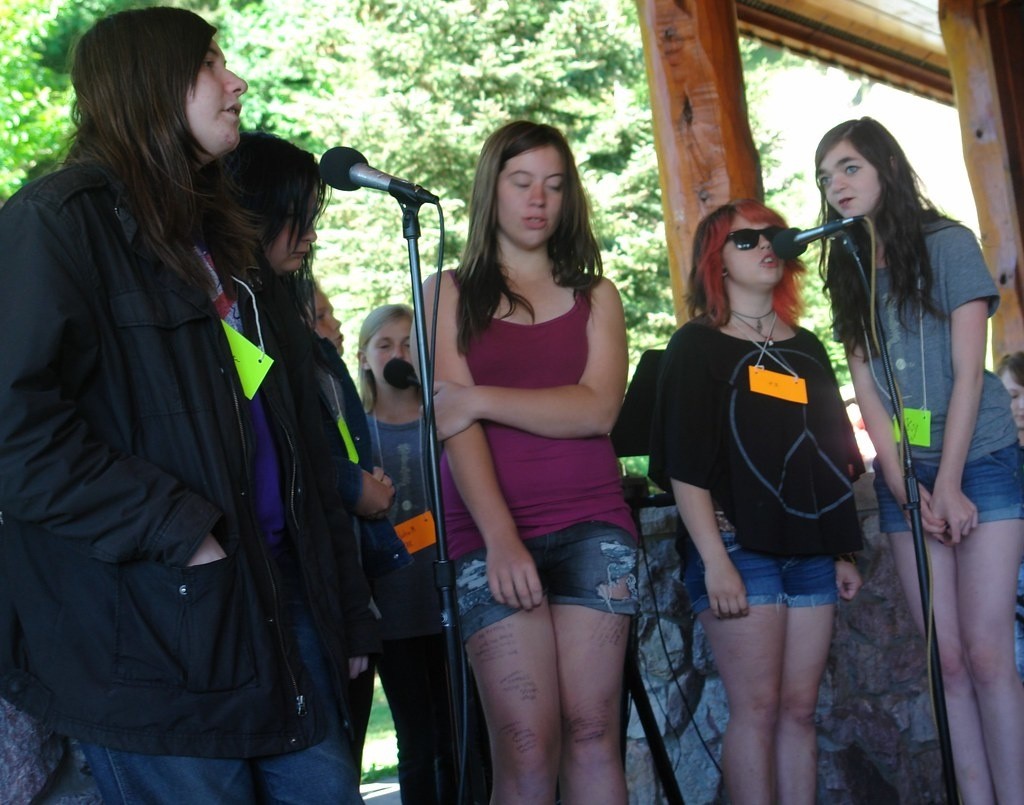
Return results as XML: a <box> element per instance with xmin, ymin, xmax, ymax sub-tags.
<box><xmin>319</xmin><ymin>146</ymin><xmax>441</xmax><ymax>203</ymax></box>
<box><xmin>772</xmin><ymin>215</ymin><xmax>863</xmax><ymax>260</ymax></box>
<box><xmin>383</xmin><ymin>358</ymin><xmax>422</xmax><ymax>390</ymax></box>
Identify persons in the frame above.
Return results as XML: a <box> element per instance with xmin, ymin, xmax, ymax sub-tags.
<box><xmin>197</xmin><ymin>126</ymin><xmax>396</xmax><ymax>790</ymax></box>
<box><xmin>408</xmin><ymin>119</ymin><xmax>641</xmax><ymax>805</ymax></box>
<box><xmin>352</xmin><ymin>303</ymin><xmax>492</xmax><ymax>805</ymax></box>
<box><xmin>991</xmin><ymin>345</ymin><xmax>1024</xmax><ymax>679</ymax></box>
<box><xmin>653</xmin><ymin>196</ymin><xmax>868</xmax><ymax>805</ymax></box>
<box><xmin>815</xmin><ymin>114</ymin><xmax>1024</xmax><ymax>805</ymax></box>
<box><xmin>0</xmin><ymin>6</ymin><xmax>370</xmax><ymax>805</ymax></box>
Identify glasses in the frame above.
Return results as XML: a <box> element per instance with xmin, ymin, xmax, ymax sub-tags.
<box><xmin>725</xmin><ymin>225</ymin><xmax>787</xmax><ymax>250</ymax></box>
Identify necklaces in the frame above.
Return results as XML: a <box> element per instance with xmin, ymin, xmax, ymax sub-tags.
<box><xmin>727</xmin><ymin>304</ymin><xmax>779</xmax><ymax>347</ymax></box>
